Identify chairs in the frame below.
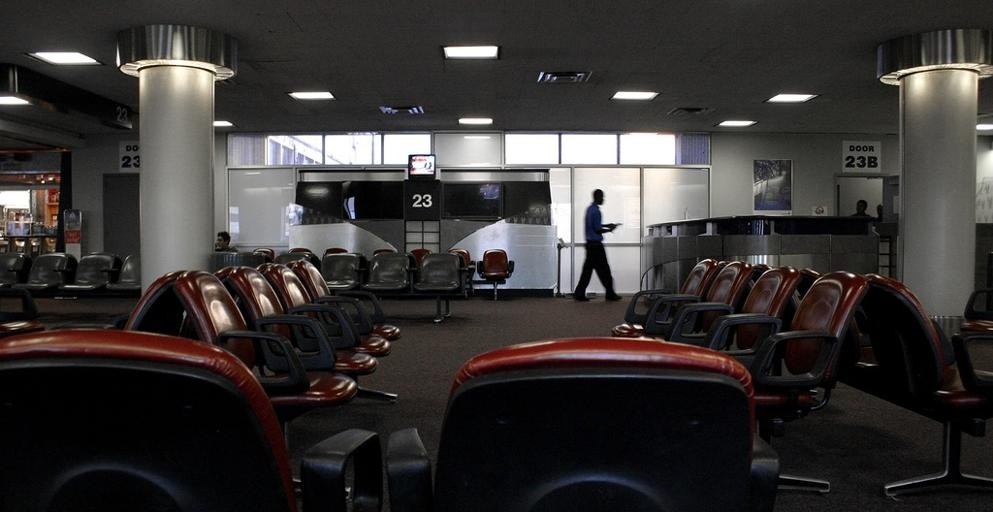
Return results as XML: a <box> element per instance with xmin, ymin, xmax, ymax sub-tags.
<box><xmin>381</xmin><ymin>339</ymin><xmax>781</xmax><ymax>510</ymax></box>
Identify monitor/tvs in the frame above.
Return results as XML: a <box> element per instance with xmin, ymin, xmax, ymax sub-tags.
<box><xmin>409</xmin><ymin>155</ymin><xmax>436</xmax><ymax>182</ymax></box>
<box><xmin>295</xmin><ymin>181</ymin><xmax>345</xmax><ymax>218</ymax></box>
<box><xmin>345</xmin><ymin>180</ymin><xmax>403</xmax><ymax>220</ymax></box>
<box><xmin>441</xmin><ymin>181</ymin><xmax>504</xmax><ymax>221</ymax></box>
<box><xmin>502</xmin><ymin>181</ymin><xmax>551</xmax><ymax>218</ymax></box>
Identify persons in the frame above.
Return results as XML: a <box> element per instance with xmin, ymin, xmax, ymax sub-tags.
<box><xmin>214</xmin><ymin>229</ymin><xmax>233</xmax><ymax>253</ymax></box>
<box><xmin>569</xmin><ymin>187</ymin><xmax>626</xmax><ymax>302</ymax></box>
<box><xmin>875</xmin><ymin>203</ymin><xmax>883</xmax><ymax>218</ymax></box>
<box><xmin>846</xmin><ymin>198</ymin><xmax>873</xmax><ymax>218</ymax></box>
